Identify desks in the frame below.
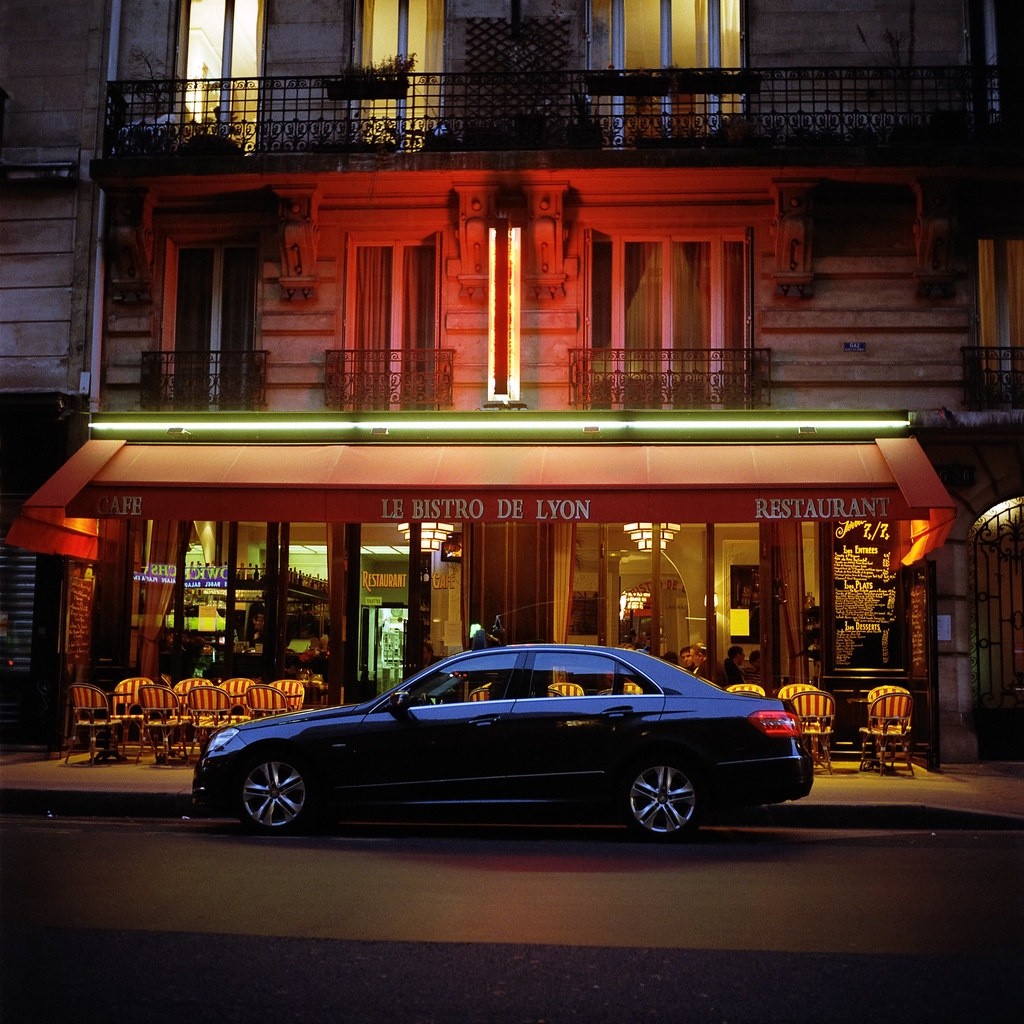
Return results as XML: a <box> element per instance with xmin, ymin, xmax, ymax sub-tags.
<box><xmin>847</xmin><ymin>698</ymin><xmax>896</xmax><ymax>772</ymax></box>
<box><xmin>85</xmin><ymin>690</ymin><xmax>133</xmax><ymax>763</ymax></box>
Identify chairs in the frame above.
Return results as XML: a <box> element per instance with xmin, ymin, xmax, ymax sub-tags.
<box><xmin>64</xmin><ymin>682</ymin><xmax>128</xmax><ymax>767</ymax></box>
<box><xmin>135</xmin><ymin>677</ymin><xmax>307</xmax><ymax>766</ymax></box>
<box><xmin>470</xmin><ymin>679</ymin><xmax>643</xmax><ymax>705</ymax></box>
<box><xmin>859</xmin><ymin>692</ymin><xmax>916</xmax><ymax>776</ymax></box>
<box><xmin>726</xmin><ymin>683</ymin><xmax>817</xmax><ymax>700</ymax></box>
<box><xmin>867</xmin><ymin>685</ymin><xmax>911</xmax><ymax>769</ymax></box>
<box><xmin>106</xmin><ymin>677</ymin><xmax>156</xmax><ymax>761</ymax></box>
<box><xmin>790</xmin><ymin>691</ymin><xmax>837</xmax><ymax>776</ymax></box>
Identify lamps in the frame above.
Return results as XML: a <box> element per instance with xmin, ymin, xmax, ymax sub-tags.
<box><xmin>623</xmin><ymin>523</ymin><xmax>682</xmax><ymax>554</ymax></box>
<box><xmin>622</xmin><ymin>591</ymin><xmax>652</xmax><ymax>611</ymax></box>
<box><xmin>398</xmin><ymin>522</ymin><xmax>454</xmax><ymax>553</ymax></box>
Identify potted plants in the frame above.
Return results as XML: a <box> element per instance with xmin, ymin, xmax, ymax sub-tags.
<box><xmin>673</xmin><ymin>69</ymin><xmax>763</xmax><ymax>95</ymax></box>
<box><xmin>586</xmin><ymin>65</ymin><xmax>671</xmax><ymax>97</ymax></box>
<box><xmin>326</xmin><ymin>52</ymin><xmax>417</xmax><ymax>101</ymax></box>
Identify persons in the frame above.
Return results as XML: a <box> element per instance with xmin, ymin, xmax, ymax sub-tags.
<box><xmin>662</xmin><ymin>641</ymin><xmax>763</xmax><ymax>693</ymax></box>
<box><xmin>420</xmin><ymin>640</ymin><xmax>443</xmax><ymax>670</ymax></box>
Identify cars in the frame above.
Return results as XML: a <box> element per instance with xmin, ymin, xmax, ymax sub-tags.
<box><xmin>189</xmin><ymin>628</ymin><xmax>816</xmax><ymax>835</ymax></box>
